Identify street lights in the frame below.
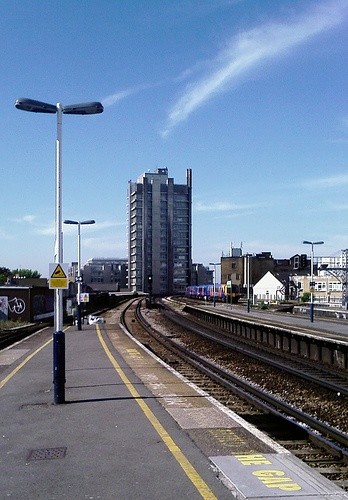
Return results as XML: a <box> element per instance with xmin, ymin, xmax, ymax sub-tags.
<box><xmin>244</xmin><ymin>254</ymin><xmax>253</xmax><ymax>312</ymax></box>
<box><xmin>14</xmin><ymin>96</ymin><xmax>105</xmax><ymax>404</ymax></box>
<box><xmin>302</xmin><ymin>240</ymin><xmax>324</xmax><ymax>323</ymax></box>
<box><xmin>63</xmin><ymin>219</ymin><xmax>95</xmax><ymax>330</ymax></box>
<box><xmin>209</xmin><ymin>262</ymin><xmax>222</xmax><ymax>307</ymax></box>
